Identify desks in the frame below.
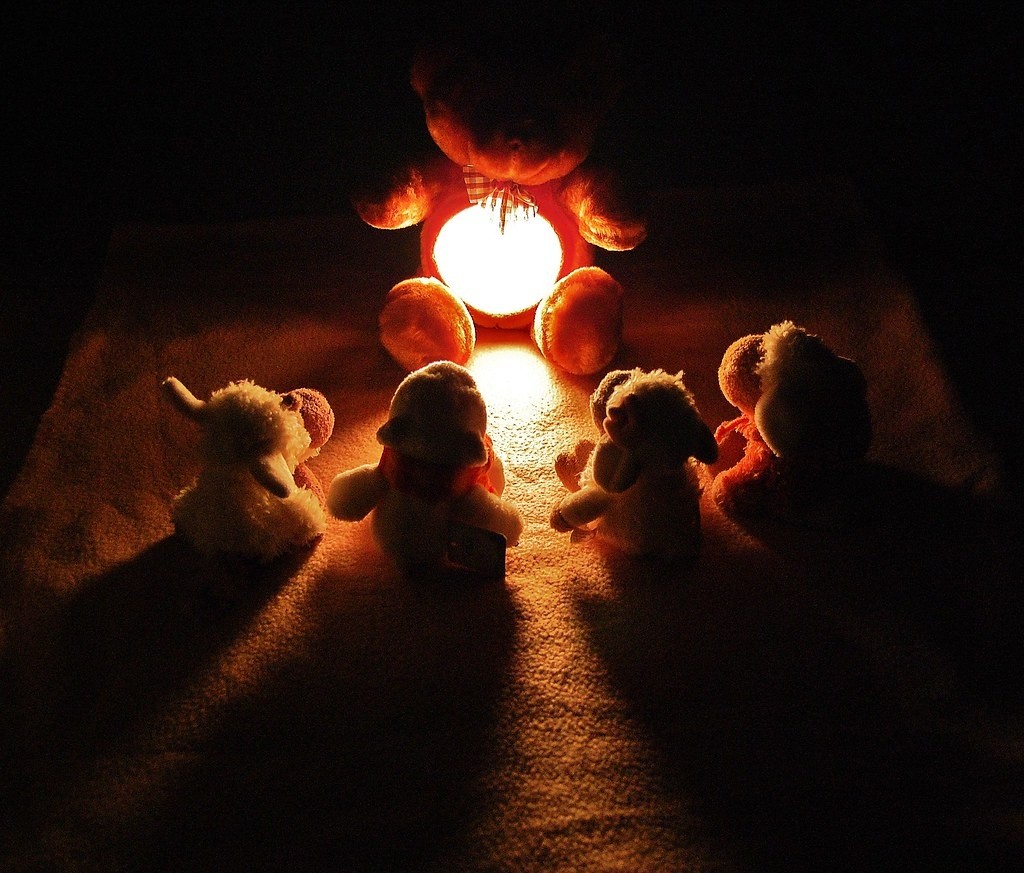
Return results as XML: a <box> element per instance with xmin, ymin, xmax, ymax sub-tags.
<box><xmin>0</xmin><ymin>181</ymin><xmax>1024</xmax><ymax>873</ymax></box>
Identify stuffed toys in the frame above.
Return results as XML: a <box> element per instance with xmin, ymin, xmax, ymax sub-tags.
<box><xmin>704</xmin><ymin>319</ymin><xmax>871</xmax><ymax>522</ymax></box>
<box><xmin>327</xmin><ymin>359</ymin><xmax>525</xmax><ymax>587</ymax></box>
<box><xmin>160</xmin><ymin>376</ymin><xmax>334</xmax><ymax>574</ymax></box>
<box><xmin>351</xmin><ymin>37</ymin><xmax>652</xmax><ymax>379</ymax></box>
<box><xmin>549</xmin><ymin>366</ymin><xmax>720</xmax><ymax>564</ymax></box>
